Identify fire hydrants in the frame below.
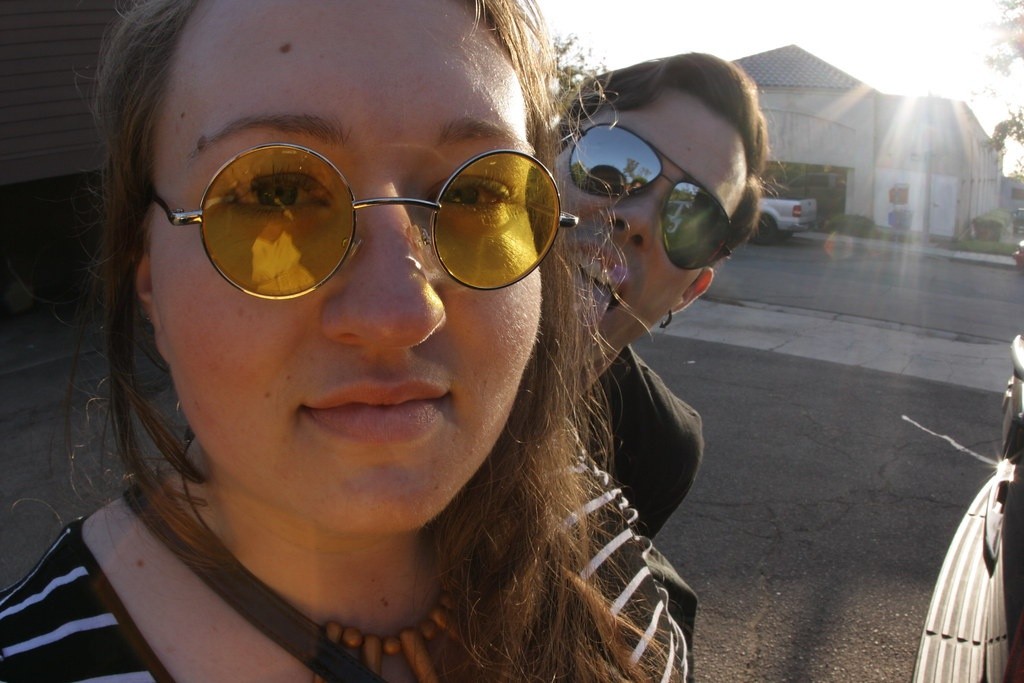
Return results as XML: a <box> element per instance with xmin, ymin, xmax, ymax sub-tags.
<box><xmin>1013</xmin><ymin>240</ymin><xmax>1024</xmax><ymax>267</ymax></box>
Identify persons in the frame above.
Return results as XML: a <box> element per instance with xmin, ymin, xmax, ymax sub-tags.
<box><xmin>581</xmin><ymin>165</ymin><xmax>643</xmax><ymax>195</ymax></box>
<box><xmin>0</xmin><ymin>0</ymin><xmax>697</xmax><ymax>683</ymax></box>
<box><xmin>524</xmin><ymin>53</ymin><xmax>768</xmax><ymax>545</ymax></box>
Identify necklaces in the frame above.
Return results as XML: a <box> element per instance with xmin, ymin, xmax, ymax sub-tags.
<box><xmin>183</xmin><ymin>433</ymin><xmax>486</xmax><ymax>683</ymax></box>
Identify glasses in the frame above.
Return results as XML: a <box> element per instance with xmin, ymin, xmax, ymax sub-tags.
<box><xmin>561</xmin><ymin>123</ymin><xmax>731</xmax><ymax>270</ymax></box>
<box><xmin>144</xmin><ymin>142</ymin><xmax>578</xmax><ymax>300</ymax></box>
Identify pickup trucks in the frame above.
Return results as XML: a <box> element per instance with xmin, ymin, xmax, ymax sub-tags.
<box><xmin>751</xmin><ymin>195</ymin><xmax>818</xmax><ymax>246</ymax></box>
